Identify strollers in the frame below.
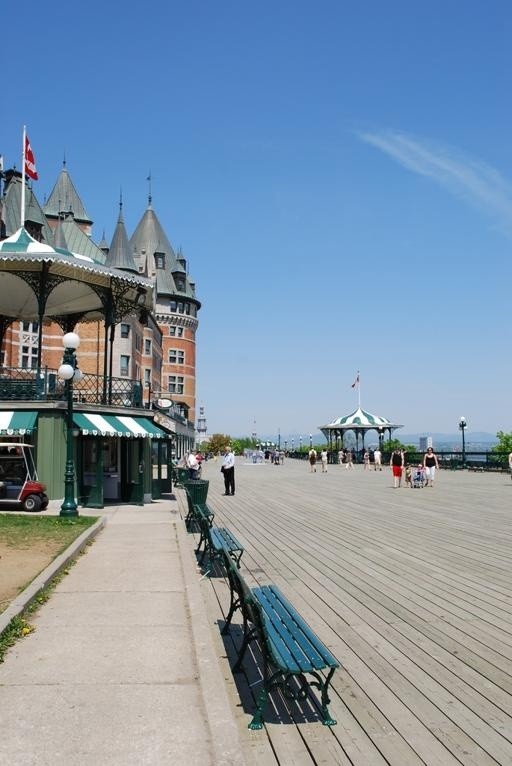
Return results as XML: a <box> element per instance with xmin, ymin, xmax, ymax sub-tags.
<box><xmin>411</xmin><ymin>469</ymin><xmax>425</xmax><ymax>488</ymax></box>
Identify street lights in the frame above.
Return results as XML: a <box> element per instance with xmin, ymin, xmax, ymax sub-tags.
<box><xmin>457</xmin><ymin>416</ymin><xmax>469</xmax><ymax>461</ymax></box>
<box><xmin>57</xmin><ymin>331</ymin><xmax>84</xmax><ymax>520</ymax></box>
<box><xmin>285</xmin><ymin>433</ymin><xmax>313</xmax><ymax>451</ymax></box>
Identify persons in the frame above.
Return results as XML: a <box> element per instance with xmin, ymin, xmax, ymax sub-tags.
<box><xmin>171</xmin><ymin>446</ymin><xmax>439</xmax><ymax>489</ymax></box>
<box><xmin>222</xmin><ymin>447</ymin><xmax>235</xmax><ymax>495</ymax></box>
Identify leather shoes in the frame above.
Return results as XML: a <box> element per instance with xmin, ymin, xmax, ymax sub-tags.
<box><xmin>222</xmin><ymin>493</ymin><xmax>235</xmax><ymax>496</ymax></box>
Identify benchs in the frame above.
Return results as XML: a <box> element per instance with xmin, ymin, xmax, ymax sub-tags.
<box><xmin>190</xmin><ymin>502</ymin><xmax>245</xmax><ymax>576</ymax></box>
<box><xmin>216</xmin><ymin>545</ymin><xmax>340</xmax><ymax>730</ymax></box>
<box><xmin>182</xmin><ymin>486</ymin><xmax>215</xmax><ymax>530</ymax></box>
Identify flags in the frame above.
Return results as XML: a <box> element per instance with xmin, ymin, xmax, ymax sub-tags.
<box><xmin>25</xmin><ymin>136</ymin><xmax>38</xmax><ymax>180</ymax></box>
<box><xmin>352</xmin><ymin>370</ymin><xmax>359</xmax><ymax>392</ymax></box>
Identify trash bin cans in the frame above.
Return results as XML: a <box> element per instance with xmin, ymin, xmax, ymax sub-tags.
<box><xmin>183</xmin><ymin>479</ymin><xmax>209</xmax><ymax>518</ymax></box>
